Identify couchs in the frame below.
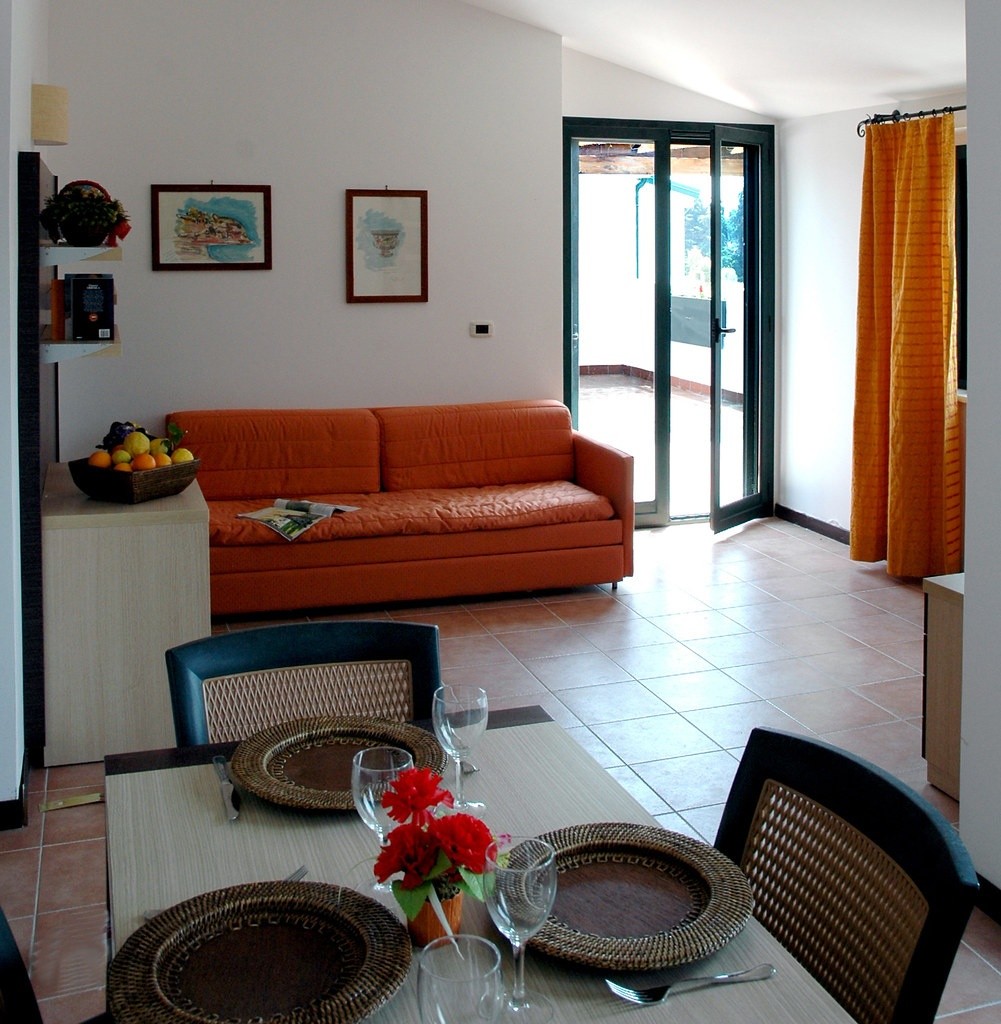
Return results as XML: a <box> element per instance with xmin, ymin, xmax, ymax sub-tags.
<box><xmin>166</xmin><ymin>398</ymin><xmax>634</xmax><ymax>616</ymax></box>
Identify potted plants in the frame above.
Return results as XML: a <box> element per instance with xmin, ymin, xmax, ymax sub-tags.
<box><xmin>41</xmin><ymin>181</ymin><xmax>130</xmax><ymax>246</ymax></box>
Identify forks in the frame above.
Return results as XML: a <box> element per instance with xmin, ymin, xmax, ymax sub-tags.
<box><xmin>605</xmin><ymin>963</ymin><xmax>775</xmax><ymax>1007</ymax></box>
<box><xmin>459</xmin><ymin>758</ymin><xmax>481</xmax><ymax>774</ymax></box>
<box><xmin>142</xmin><ymin>866</ymin><xmax>311</xmax><ymax>921</ymax></box>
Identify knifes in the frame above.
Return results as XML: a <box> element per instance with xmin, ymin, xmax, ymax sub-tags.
<box><xmin>212</xmin><ymin>755</ymin><xmax>240</xmax><ymax>821</ymax></box>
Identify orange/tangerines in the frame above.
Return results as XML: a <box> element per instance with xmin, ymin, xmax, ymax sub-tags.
<box><xmin>90</xmin><ymin>445</ymin><xmax>170</xmax><ymax>473</ymax></box>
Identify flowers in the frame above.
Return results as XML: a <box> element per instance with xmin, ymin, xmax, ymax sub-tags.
<box><xmin>374</xmin><ymin>767</ymin><xmax>510</xmax><ymax>920</ymax></box>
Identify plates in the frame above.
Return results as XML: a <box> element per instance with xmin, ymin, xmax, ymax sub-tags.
<box><xmin>230</xmin><ymin>715</ymin><xmax>448</xmax><ymax>812</ymax></box>
<box><xmin>106</xmin><ymin>879</ymin><xmax>412</xmax><ymax>1024</ymax></box>
<box><xmin>492</xmin><ymin>821</ymin><xmax>755</xmax><ymax>971</ymax></box>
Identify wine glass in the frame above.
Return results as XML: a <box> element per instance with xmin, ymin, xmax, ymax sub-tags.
<box><xmin>418</xmin><ymin>933</ymin><xmax>504</xmax><ymax>1024</ymax></box>
<box><xmin>432</xmin><ymin>683</ymin><xmax>488</xmax><ymax>819</ymax></box>
<box><xmin>352</xmin><ymin>748</ymin><xmax>417</xmax><ymax>913</ymax></box>
<box><xmin>476</xmin><ymin>836</ymin><xmax>557</xmax><ymax>1024</ymax></box>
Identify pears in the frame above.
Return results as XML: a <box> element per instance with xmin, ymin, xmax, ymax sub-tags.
<box><xmin>114</xmin><ymin>433</ymin><xmax>191</xmax><ymax>465</ymax></box>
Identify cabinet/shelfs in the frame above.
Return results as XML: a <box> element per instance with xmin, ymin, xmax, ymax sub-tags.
<box><xmin>41</xmin><ymin>463</ymin><xmax>212</xmax><ymax>766</ymax></box>
<box><xmin>42</xmin><ymin>244</ymin><xmax>119</xmax><ymax>361</ymax></box>
<box><xmin>922</xmin><ymin>573</ymin><xmax>964</xmax><ymax>801</ymax></box>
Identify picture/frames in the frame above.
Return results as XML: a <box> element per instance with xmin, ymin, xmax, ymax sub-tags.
<box><xmin>346</xmin><ymin>188</ymin><xmax>429</xmax><ymax>303</ymax></box>
<box><xmin>151</xmin><ymin>184</ymin><xmax>272</xmax><ymax>271</ymax></box>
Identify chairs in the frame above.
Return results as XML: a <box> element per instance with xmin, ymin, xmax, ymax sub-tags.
<box><xmin>165</xmin><ymin>620</ymin><xmax>444</xmax><ymax>747</ymax></box>
<box><xmin>714</xmin><ymin>726</ymin><xmax>981</xmax><ymax>1024</ymax></box>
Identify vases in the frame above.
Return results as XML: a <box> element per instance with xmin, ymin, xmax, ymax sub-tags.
<box><xmin>409</xmin><ymin>888</ymin><xmax>464</xmax><ymax>948</ymax></box>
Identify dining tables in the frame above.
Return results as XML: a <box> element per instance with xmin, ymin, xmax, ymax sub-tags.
<box><xmin>105</xmin><ymin>705</ymin><xmax>857</xmax><ymax>1024</ymax></box>
